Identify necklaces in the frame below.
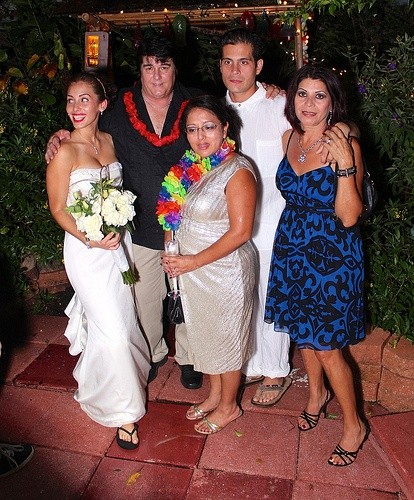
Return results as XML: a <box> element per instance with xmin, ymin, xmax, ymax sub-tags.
<box><xmin>297</xmin><ymin>135</ymin><xmax>324</xmax><ymax>163</ymax></box>
<box><xmin>124</xmin><ymin>91</ymin><xmax>188</xmax><ymax>147</ymax></box>
<box><xmin>76</xmin><ymin>129</ymin><xmax>99</xmax><ymax>155</ymax></box>
<box><xmin>156</xmin><ymin>137</ymin><xmax>235</xmax><ymax>231</ymax></box>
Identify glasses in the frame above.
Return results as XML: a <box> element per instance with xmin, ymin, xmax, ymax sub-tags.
<box><xmin>185</xmin><ymin>123</ymin><xmax>225</xmax><ymax>134</ymax></box>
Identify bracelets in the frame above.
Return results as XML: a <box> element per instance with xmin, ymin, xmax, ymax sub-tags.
<box><xmin>337</xmin><ymin>166</ymin><xmax>357</xmax><ymax>177</ymax></box>
<box><xmin>86</xmin><ymin>240</ymin><xmax>91</xmax><ymax>249</ymax></box>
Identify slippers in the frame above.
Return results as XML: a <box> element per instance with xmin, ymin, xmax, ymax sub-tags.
<box><xmin>116</xmin><ymin>423</ymin><xmax>139</xmax><ymax>449</ymax></box>
<box><xmin>239</xmin><ymin>374</ymin><xmax>265</xmax><ymax>389</ymax></box>
<box><xmin>251</xmin><ymin>376</ymin><xmax>292</xmax><ymax>408</ymax></box>
<box><xmin>194</xmin><ymin>409</ymin><xmax>243</xmax><ymax>434</ymax></box>
<box><xmin>186</xmin><ymin>404</ymin><xmax>215</xmax><ymax>420</ymax></box>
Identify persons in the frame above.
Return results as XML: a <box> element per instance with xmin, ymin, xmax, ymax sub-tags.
<box><xmin>263</xmin><ymin>63</ymin><xmax>372</xmax><ymax>466</ymax></box>
<box><xmin>46</xmin><ymin>72</ymin><xmax>151</xmax><ymax>450</ymax></box>
<box><xmin>216</xmin><ymin>26</ymin><xmax>364</xmax><ymax>406</ymax></box>
<box><xmin>157</xmin><ymin>94</ymin><xmax>258</xmax><ymax>435</ymax></box>
<box><xmin>44</xmin><ymin>33</ymin><xmax>286</xmax><ymax>387</ymax></box>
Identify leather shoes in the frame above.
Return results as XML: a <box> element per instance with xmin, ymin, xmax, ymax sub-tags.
<box><xmin>180</xmin><ymin>364</ymin><xmax>202</xmax><ymax>389</ymax></box>
<box><xmin>148</xmin><ymin>355</ymin><xmax>168</xmax><ymax>382</ymax></box>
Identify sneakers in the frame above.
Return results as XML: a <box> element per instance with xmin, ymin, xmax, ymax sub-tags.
<box><xmin>0</xmin><ymin>442</ymin><xmax>34</xmax><ymax>477</ymax></box>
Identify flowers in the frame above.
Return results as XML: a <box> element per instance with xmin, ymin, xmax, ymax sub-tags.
<box><xmin>65</xmin><ymin>175</ymin><xmax>138</xmax><ymax>286</ymax></box>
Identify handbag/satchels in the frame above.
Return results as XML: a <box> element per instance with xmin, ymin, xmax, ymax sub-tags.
<box><xmin>167</xmin><ymin>276</ymin><xmax>185</xmax><ymax>323</ymax></box>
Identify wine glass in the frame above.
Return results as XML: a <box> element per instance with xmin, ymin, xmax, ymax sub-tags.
<box><xmin>164</xmin><ymin>240</ymin><xmax>186</xmax><ymax>297</ymax></box>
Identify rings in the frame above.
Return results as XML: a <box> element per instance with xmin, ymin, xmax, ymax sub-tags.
<box><xmin>326</xmin><ymin>139</ymin><xmax>331</xmax><ymax>144</ymax></box>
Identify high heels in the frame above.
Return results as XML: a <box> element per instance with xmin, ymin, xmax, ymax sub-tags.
<box><xmin>328</xmin><ymin>425</ymin><xmax>370</xmax><ymax>466</ymax></box>
<box><xmin>298</xmin><ymin>390</ymin><xmax>332</xmax><ymax>431</ymax></box>
